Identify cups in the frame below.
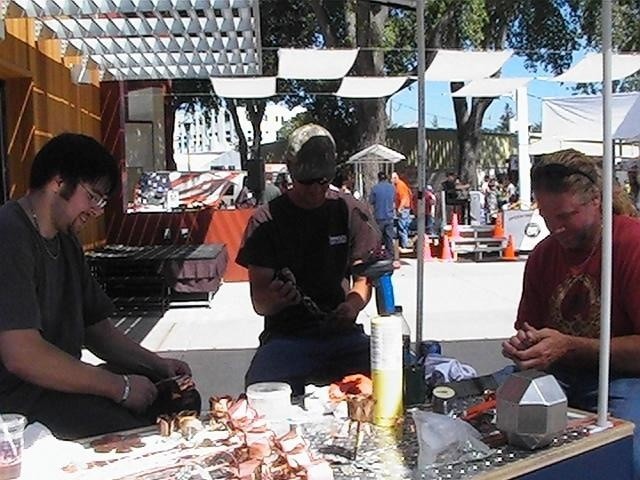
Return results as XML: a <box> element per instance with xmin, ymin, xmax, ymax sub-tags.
<box><xmin>0</xmin><ymin>414</ymin><xmax>28</xmax><ymax>480</ymax></box>
<box><xmin>247</xmin><ymin>381</ymin><xmax>294</xmax><ymax>423</ymax></box>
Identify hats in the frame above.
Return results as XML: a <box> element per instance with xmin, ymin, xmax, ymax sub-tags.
<box><xmin>283</xmin><ymin>122</ymin><xmax>337</xmax><ymax>181</ymax></box>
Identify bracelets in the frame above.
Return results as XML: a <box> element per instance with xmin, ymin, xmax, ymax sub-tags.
<box><xmin>119</xmin><ymin>370</ymin><xmax>132</xmax><ymax>408</ymax></box>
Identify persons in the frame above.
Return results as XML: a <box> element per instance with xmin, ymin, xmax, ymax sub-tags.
<box><xmin>500</xmin><ymin>148</ymin><xmax>640</xmax><ymax>480</ymax></box>
<box><xmin>233</xmin><ymin>119</ymin><xmax>417</xmax><ymax>402</ymax></box>
<box><xmin>234</xmin><ymin>168</ymin><xmax>291</xmax><ymax>208</ymax></box>
<box><xmin>1</xmin><ymin>132</ymin><xmax>202</xmax><ymax>441</ymax></box>
<box><xmin>369</xmin><ymin>169</ymin><xmax>520</xmax><ymax>265</ymax></box>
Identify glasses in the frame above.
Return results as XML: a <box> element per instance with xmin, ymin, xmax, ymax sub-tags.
<box><xmin>295</xmin><ymin>177</ymin><xmax>331</xmax><ymax>186</ymax></box>
<box><xmin>80</xmin><ymin>184</ymin><xmax>108</xmax><ymax>209</ymax></box>
<box><xmin>534</xmin><ymin>163</ymin><xmax>596</xmax><ymax>184</ymax></box>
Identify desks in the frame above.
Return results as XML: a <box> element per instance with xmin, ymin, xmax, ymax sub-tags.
<box><xmin>126</xmin><ymin>207</ymin><xmax>258</xmax><ymax>283</ymax></box>
<box><xmin>73</xmin><ymin>383</ymin><xmax>637</xmax><ymax>480</ymax></box>
<box><xmin>85</xmin><ymin>243</ymin><xmax>229</xmax><ymax>317</ymax></box>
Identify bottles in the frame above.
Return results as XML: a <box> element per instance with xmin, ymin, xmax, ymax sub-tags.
<box><xmin>390</xmin><ymin>304</ymin><xmax>414</xmax><ymax>406</ymax></box>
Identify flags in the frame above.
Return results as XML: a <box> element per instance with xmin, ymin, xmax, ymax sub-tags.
<box><xmin>128</xmin><ymin>171</ymin><xmax>237</xmax><ymax>213</ymax></box>
